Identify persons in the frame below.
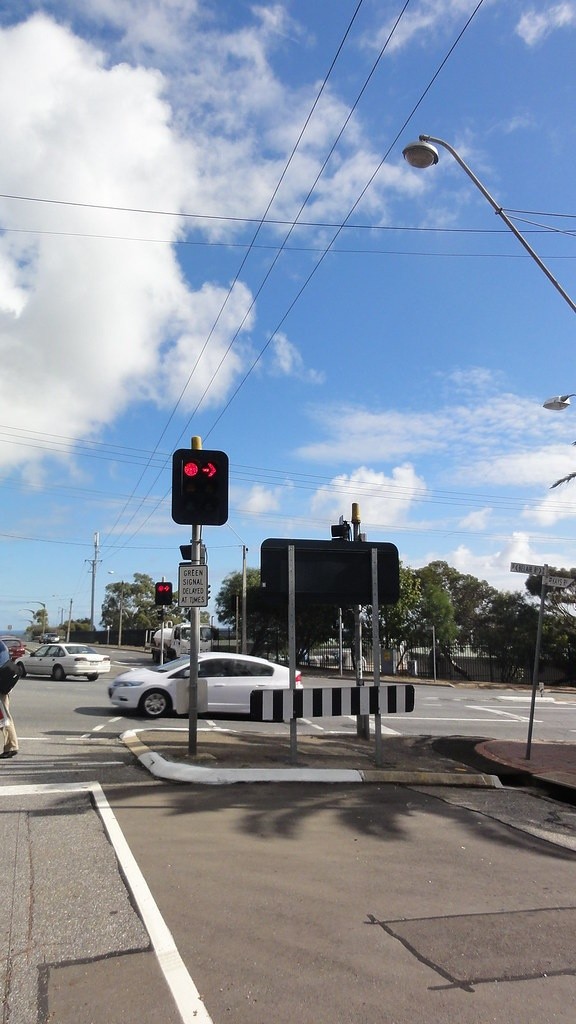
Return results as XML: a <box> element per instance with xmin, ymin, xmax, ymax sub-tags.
<box><xmin>0</xmin><ymin>640</ymin><xmax>18</xmax><ymax>758</ymax></box>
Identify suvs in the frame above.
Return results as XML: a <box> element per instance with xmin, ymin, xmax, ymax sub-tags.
<box><xmin>39</xmin><ymin>633</ymin><xmax>59</xmax><ymax>645</ymax></box>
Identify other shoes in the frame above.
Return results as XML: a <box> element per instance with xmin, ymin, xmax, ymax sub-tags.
<box><xmin>0</xmin><ymin>751</ymin><xmax>18</xmax><ymax>758</ymax></box>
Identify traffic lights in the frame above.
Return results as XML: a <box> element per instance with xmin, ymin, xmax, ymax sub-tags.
<box><xmin>332</xmin><ymin>521</ymin><xmax>352</xmax><ymax>543</ymax></box>
<box><xmin>172</xmin><ymin>449</ymin><xmax>228</xmax><ymax>526</ymax></box>
<box><xmin>155</xmin><ymin>582</ymin><xmax>173</xmax><ymax>605</ymax></box>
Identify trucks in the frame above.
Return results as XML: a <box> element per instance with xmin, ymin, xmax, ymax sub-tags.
<box><xmin>150</xmin><ymin>623</ymin><xmax>219</xmax><ymax>663</ymax></box>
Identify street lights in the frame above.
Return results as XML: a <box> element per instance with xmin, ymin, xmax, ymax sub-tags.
<box><xmin>108</xmin><ymin>571</ymin><xmax>124</xmax><ymax>648</ymax></box>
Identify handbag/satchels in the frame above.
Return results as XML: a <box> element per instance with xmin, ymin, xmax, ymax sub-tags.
<box><xmin>0</xmin><ymin>657</ymin><xmax>22</xmax><ymax>694</ymax></box>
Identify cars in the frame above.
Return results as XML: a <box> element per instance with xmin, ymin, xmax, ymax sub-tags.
<box><xmin>14</xmin><ymin>644</ymin><xmax>111</xmax><ymax>682</ymax></box>
<box><xmin>107</xmin><ymin>651</ymin><xmax>303</xmax><ymax>720</ymax></box>
<box><xmin>276</xmin><ymin>649</ymin><xmax>347</xmax><ymax>670</ymax></box>
<box><xmin>0</xmin><ymin>636</ymin><xmax>27</xmax><ymax>662</ymax></box>
